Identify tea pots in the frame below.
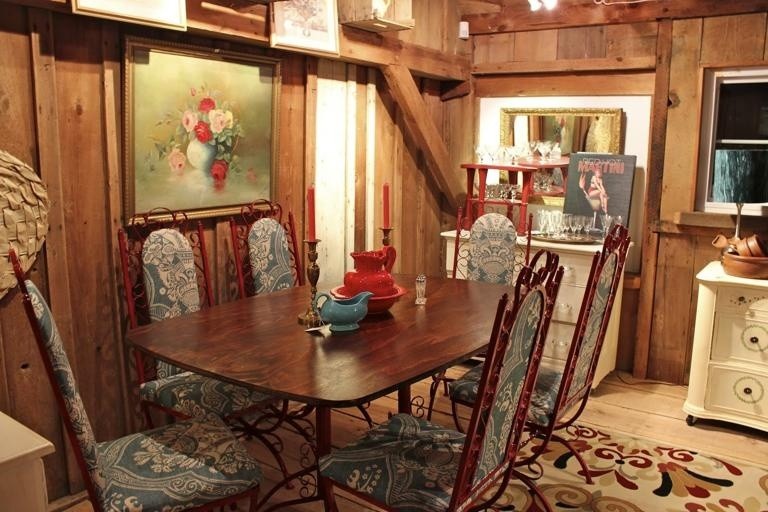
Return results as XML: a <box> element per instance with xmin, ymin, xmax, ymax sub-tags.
<box><xmin>313</xmin><ymin>291</ymin><xmax>374</xmax><ymax>336</ymax></box>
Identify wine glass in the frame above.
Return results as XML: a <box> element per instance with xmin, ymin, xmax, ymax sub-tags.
<box><xmin>475</xmin><ymin>182</ymin><xmax>520</xmax><ymax>201</ymax></box>
<box><xmin>534</xmin><ymin>210</ymin><xmax>622</xmax><ymax>241</ymax></box>
<box><xmin>533</xmin><ymin>175</ymin><xmax>553</xmax><ymax>193</ymax></box>
<box><xmin>474</xmin><ymin>138</ymin><xmax>551</xmax><ymax>166</ymax></box>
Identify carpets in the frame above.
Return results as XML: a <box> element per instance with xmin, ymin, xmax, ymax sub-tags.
<box><xmin>466</xmin><ymin>421</ymin><xmax>768</xmax><ymax>512</ymax></box>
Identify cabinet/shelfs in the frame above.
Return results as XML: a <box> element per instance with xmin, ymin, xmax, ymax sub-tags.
<box><xmin>517</xmin><ymin>157</ymin><xmax>570</xmax><ymax>198</ymax></box>
<box><xmin>681</xmin><ymin>261</ymin><xmax>768</xmax><ymax>436</ymax></box>
<box><xmin>461</xmin><ymin>163</ymin><xmax>537</xmax><ymax>236</ymax></box>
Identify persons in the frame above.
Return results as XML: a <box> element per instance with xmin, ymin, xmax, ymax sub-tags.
<box><xmin>588</xmin><ymin>167</ymin><xmax>611</xmax><ymax>215</ymax></box>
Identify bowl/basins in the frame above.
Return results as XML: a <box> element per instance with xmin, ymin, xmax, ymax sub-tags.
<box><xmin>330</xmin><ymin>286</ymin><xmax>406</xmax><ymax>314</ymax></box>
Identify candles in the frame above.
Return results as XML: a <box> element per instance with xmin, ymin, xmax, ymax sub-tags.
<box><xmin>307</xmin><ymin>187</ymin><xmax>317</xmax><ymax>240</ymax></box>
<box><xmin>382</xmin><ymin>184</ymin><xmax>390</xmax><ymax>228</ymax></box>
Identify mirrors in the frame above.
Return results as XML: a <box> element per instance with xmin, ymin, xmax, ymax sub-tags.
<box><xmin>498</xmin><ymin>107</ymin><xmax>624</xmax><ymax>213</ymax></box>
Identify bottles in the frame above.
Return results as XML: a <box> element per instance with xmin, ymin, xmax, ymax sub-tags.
<box><xmin>415</xmin><ymin>275</ymin><xmax>427</xmax><ymax>305</ymax></box>
